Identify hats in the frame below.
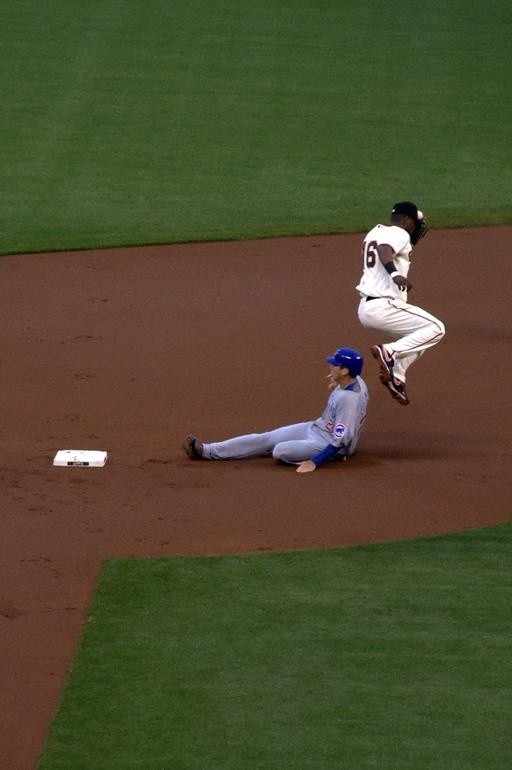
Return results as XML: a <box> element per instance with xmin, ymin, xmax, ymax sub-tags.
<box><xmin>390</xmin><ymin>201</ymin><xmax>427</xmax><ymax>226</ymax></box>
<box><xmin>324</xmin><ymin>345</ymin><xmax>363</xmax><ymax>369</ymax></box>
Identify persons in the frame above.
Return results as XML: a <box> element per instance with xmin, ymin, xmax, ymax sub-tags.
<box><xmin>181</xmin><ymin>348</ymin><xmax>370</xmax><ymax>474</ymax></box>
<box><xmin>354</xmin><ymin>199</ymin><xmax>446</xmax><ymax>404</ymax></box>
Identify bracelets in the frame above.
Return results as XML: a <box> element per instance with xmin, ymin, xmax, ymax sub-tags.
<box><xmin>389</xmin><ymin>270</ymin><xmax>401</xmax><ymax>279</ymax></box>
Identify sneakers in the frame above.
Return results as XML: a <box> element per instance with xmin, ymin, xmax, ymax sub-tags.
<box><xmin>370</xmin><ymin>343</ymin><xmax>396</xmax><ymax>380</ymax></box>
<box><xmin>377</xmin><ymin>372</ymin><xmax>410</xmax><ymax>405</ymax></box>
<box><xmin>190</xmin><ymin>436</ymin><xmax>204</xmax><ymax>460</ymax></box>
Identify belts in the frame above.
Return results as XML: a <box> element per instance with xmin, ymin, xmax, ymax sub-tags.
<box><xmin>365</xmin><ymin>295</ymin><xmax>396</xmax><ymax>304</ymax></box>
